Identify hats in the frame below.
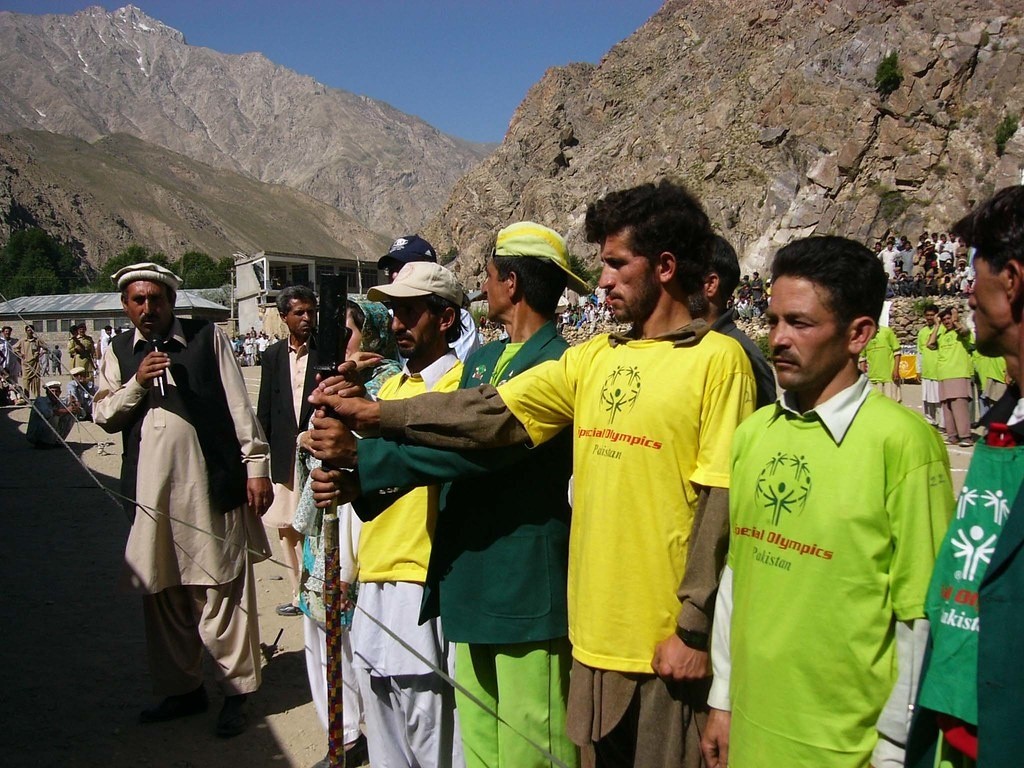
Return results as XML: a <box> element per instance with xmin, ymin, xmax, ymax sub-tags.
<box><xmin>495</xmin><ymin>221</ymin><xmax>589</xmax><ymax>294</ymax></box>
<box><xmin>43</xmin><ymin>380</ymin><xmax>62</xmax><ymax>388</ymax></box>
<box><xmin>367</xmin><ymin>262</ymin><xmax>463</xmax><ymax>307</ymax></box>
<box><xmin>69</xmin><ymin>323</ymin><xmax>86</xmax><ymax>332</ymax></box>
<box><xmin>378</xmin><ymin>236</ymin><xmax>437</xmax><ymax>271</ymax></box>
<box><xmin>70</xmin><ymin>366</ymin><xmax>85</xmax><ymax>374</ymax></box>
<box><xmin>109</xmin><ymin>262</ymin><xmax>183</xmax><ymax>292</ymax></box>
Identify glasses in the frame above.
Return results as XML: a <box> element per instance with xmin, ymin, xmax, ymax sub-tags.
<box><xmin>73</xmin><ymin>373</ymin><xmax>85</xmax><ymax>377</ymax></box>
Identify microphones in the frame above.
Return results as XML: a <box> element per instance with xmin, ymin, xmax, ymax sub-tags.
<box><xmin>151</xmin><ymin>334</ymin><xmax>166</xmax><ymax>397</ymax></box>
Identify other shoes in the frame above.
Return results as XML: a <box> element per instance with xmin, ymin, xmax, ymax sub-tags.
<box><xmin>959</xmin><ymin>437</ymin><xmax>974</xmax><ymax>446</ymax></box>
<box><xmin>216</xmin><ymin>692</ymin><xmax>257</xmax><ymax>734</ymax></box>
<box><xmin>276</xmin><ymin>603</ymin><xmax>303</xmax><ymax>615</ymax></box>
<box><xmin>345</xmin><ymin>734</ymin><xmax>367</xmax><ymax>766</ymax></box>
<box><xmin>140</xmin><ymin>684</ymin><xmax>208</xmax><ymax>721</ymax></box>
<box><xmin>926</xmin><ymin>416</ymin><xmax>939</xmax><ymax>426</ymax></box>
<box><xmin>943</xmin><ymin>435</ymin><xmax>959</xmax><ymax>444</ymax></box>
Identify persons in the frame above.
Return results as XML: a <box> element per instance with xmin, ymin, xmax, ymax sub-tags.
<box><xmin>87</xmin><ymin>263</ymin><xmax>274</xmax><ymax>738</ymax></box>
<box><xmin>858</xmin><ymin>324</ymin><xmax>901</xmax><ymax>402</ymax></box>
<box><xmin>25</xmin><ymin>381</ymin><xmax>81</xmax><ymax>449</ymax></box>
<box><xmin>0</xmin><ymin>325</ymin><xmax>49</xmax><ymax>405</ymax></box>
<box><xmin>253</xmin><ymin>284</ymin><xmax>329</xmax><ymax>617</ymax></box>
<box><xmin>67</xmin><ymin>323</ymin><xmax>124</xmax><ymax>384</ymax></box>
<box><xmin>726</xmin><ymin>272</ymin><xmax>773</xmax><ymax>325</ymax></box>
<box><xmin>914</xmin><ymin>301</ymin><xmax>1014</xmax><ymax>446</ymax></box>
<box><xmin>474</xmin><ymin>314</ymin><xmax>506</xmax><ymax>346</ymax></box>
<box><xmin>230</xmin><ymin>327</ymin><xmax>280</xmax><ymax>368</ymax></box>
<box><xmin>38</xmin><ymin>344</ymin><xmax>62</xmax><ymax>377</ymax></box>
<box><xmin>293</xmin><ymin>180</ymin><xmax>776</xmax><ymax>768</ymax></box>
<box><xmin>62</xmin><ymin>367</ymin><xmax>99</xmax><ymax>420</ymax></box>
<box><xmin>559</xmin><ymin>302</ymin><xmax>619</xmax><ymax>334</ymax></box>
<box><xmin>701</xmin><ymin>236</ymin><xmax>955</xmax><ymax>768</ymax></box>
<box><xmin>872</xmin><ymin>231</ymin><xmax>977</xmax><ymax>297</ymax></box>
<box><xmin>902</xmin><ymin>184</ymin><xmax>1024</xmax><ymax>768</ymax></box>
